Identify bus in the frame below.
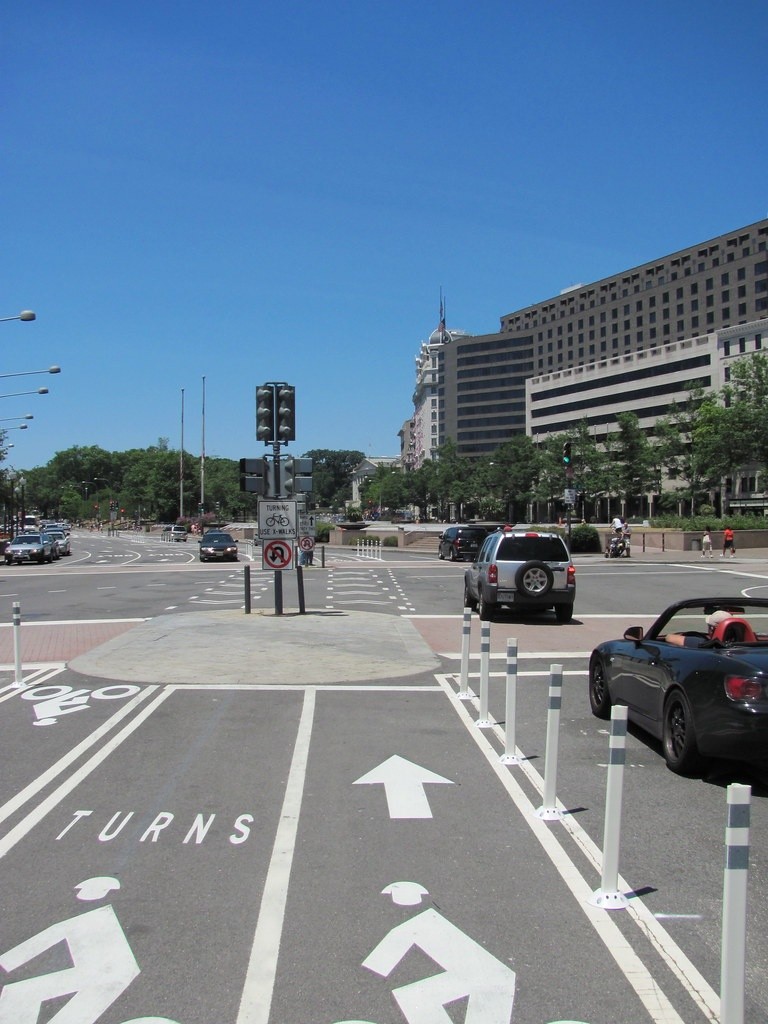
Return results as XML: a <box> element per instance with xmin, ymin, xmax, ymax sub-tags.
<box><xmin>24</xmin><ymin>515</ymin><xmax>36</xmax><ymax>531</ymax></box>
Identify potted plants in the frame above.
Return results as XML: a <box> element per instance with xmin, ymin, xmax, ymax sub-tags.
<box><xmin>344</xmin><ymin>506</ymin><xmax>363</xmax><ymax>523</ymax></box>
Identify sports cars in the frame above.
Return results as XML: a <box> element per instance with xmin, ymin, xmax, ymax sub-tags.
<box><xmin>587</xmin><ymin>598</ymin><xmax>768</xmax><ymax>770</ymax></box>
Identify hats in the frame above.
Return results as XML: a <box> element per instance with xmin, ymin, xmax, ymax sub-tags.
<box><xmin>705</xmin><ymin>610</ymin><xmax>732</xmax><ymax>628</ymax></box>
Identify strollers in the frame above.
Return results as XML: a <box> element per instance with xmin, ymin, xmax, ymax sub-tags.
<box><xmin>605</xmin><ymin>537</ymin><xmax>625</xmax><ymax>558</ymax></box>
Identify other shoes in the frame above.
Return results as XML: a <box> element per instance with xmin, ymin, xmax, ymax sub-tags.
<box><xmin>709</xmin><ymin>555</ymin><xmax>712</xmax><ymax>558</ymax></box>
<box><xmin>729</xmin><ymin>555</ymin><xmax>734</xmax><ymax>558</ymax></box>
<box><xmin>701</xmin><ymin>555</ymin><xmax>705</xmax><ymax>558</ymax></box>
<box><xmin>720</xmin><ymin>554</ymin><xmax>725</xmax><ymax>557</ymax></box>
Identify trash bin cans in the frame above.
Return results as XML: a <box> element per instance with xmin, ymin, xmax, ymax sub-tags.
<box><xmin>691</xmin><ymin>538</ymin><xmax>701</xmax><ymax>550</ymax></box>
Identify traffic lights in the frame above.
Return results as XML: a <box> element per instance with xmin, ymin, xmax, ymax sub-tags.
<box><xmin>292</xmin><ymin>457</ymin><xmax>313</xmax><ymax>493</ymax></box>
<box><xmin>563</xmin><ymin>442</ymin><xmax>571</xmax><ymax>467</ymax></box>
<box><xmin>121</xmin><ymin>507</ymin><xmax>123</xmax><ymax>512</ymax></box>
<box><xmin>198</xmin><ymin>503</ymin><xmax>203</xmax><ymax>513</ymax></box>
<box><xmin>240</xmin><ymin>457</ymin><xmax>267</xmax><ymax>496</ymax></box>
<box><xmin>109</xmin><ymin>500</ymin><xmax>118</xmax><ymax>511</ymax></box>
<box><xmin>216</xmin><ymin>501</ymin><xmax>219</xmax><ymax>510</ymax></box>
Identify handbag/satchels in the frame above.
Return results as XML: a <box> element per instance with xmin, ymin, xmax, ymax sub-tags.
<box><xmin>703</xmin><ymin>531</ymin><xmax>711</xmax><ymax>544</ymax></box>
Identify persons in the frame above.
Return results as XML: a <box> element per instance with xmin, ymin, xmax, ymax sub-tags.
<box><xmin>298</xmin><ymin>543</ymin><xmax>316</xmax><ymax>566</ymax></box>
<box><xmin>254</xmin><ymin>533</ymin><xmax>259</xmax><ymax>546</ymax></box>
<box><xmin>701</xmin><ymin>525</ymin><xmax>714</xmax><ymax>558</ymax></box>
<box><xmin>609</xmin><ymin>516</ymin><xmax>631</xmax><ymax>558</ymax></box>
<box><xmin>666</xmin><ymin>610</ymin><xmax>733</xmax><ymax>648</ymax></box>
<box><xmin>720</xmin><ymin>526</ymin><xmax>736</xmax><ymax>557</ymax></box>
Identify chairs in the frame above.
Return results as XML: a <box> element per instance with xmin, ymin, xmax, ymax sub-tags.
<box><xmin>712</xmin><ymin>618</ymin><xmax>757</xmax><ymax>642</ymax></box>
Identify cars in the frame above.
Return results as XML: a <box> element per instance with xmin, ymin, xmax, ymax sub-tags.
<box><xmin>28</xmin><ymin>520</ymin><xmax>75</xmax><ymax>560</ymax></box>
<box><xmin>329</xmin><ymin>515</ymin><xmax>344</xmax><ymax>524</ymax></box>
<box><xmin>198</xmin><ymin>529</ymin><xmax>240</xmax><ymax>562</ymax></box>
<box><xmin>0</xmin><ymin>524</ymin><xmax>22</xmax><ymax>533</ymax></box>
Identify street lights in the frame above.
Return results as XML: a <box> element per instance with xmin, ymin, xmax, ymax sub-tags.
<box><xmin>9</xmin><ymin>471</ymin><xmax>26</xmax><ymax>538</ymax></box>
<box><xmin>94</xmin><ymin>478</ymin><xmax>114</xmax><ymax>536</ymax></box>
<box><xmin>82</xmin><ymin>481</ymin><xmax>99</xmax><ymax>531</ymax></box>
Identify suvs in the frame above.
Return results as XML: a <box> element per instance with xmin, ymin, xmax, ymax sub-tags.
<box><xmin>161</xmin><ymin>526</ymin><xmax>187</xmax><ymax>542</ymax></box>
<box><xmin>464</xmin><ymin>528</ymin><xmax>576</xmax><ymax>622</ymax></box>
<box><xmin>437</xmin><ymin>526</ymin><xmax>489</xmax><ymax>561</ymax></box>
<box><xmin>5</xmin><ymin>533</ymin><xmax>52</xmax><ymax>564</ymax></box>
